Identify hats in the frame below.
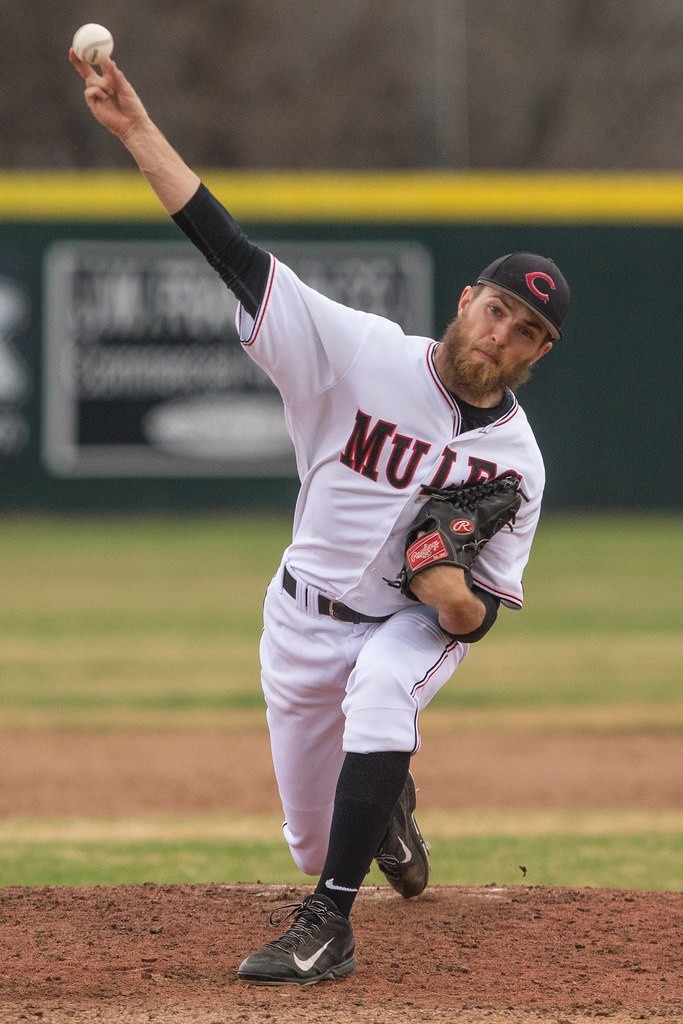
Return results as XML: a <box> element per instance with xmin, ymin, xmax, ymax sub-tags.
<box><xmin>473</xmin><ymin>253</ymin><xmax>570</xmax><ymax>341</ymax></box>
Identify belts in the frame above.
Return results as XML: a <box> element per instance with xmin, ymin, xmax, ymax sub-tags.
<box><xmin>282</xmin><ymin>566</ymin><xmax>395</xmax><ymax>622</ymax></box>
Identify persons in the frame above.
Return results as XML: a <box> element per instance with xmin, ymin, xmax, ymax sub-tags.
<box><xmin>66</xmin><ymin>47</ymin><xmax>571</xmax><ymax>983</ymax></box>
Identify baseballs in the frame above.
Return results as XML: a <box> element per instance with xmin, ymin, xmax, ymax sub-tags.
<box><xmin>72</xmin><ymin>23</ymin><xmax>114</xmax><ymax>64</ymax></box>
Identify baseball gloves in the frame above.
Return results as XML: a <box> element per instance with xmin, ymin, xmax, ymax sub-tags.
<box><xmin>400</xmin><ymin>474</ymin><xmax>523</xmax><ymax>603</ymax></box>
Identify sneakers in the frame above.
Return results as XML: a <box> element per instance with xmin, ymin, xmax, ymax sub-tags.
<box><xmin>374</xmin><ymin>771</ymin><xmax>430</xmax><ymax>898</ymax></box>
<box><xmin>239</xmin><ymin>894</ymin><xmax>355</xmax><ymax>987</ymax></box>
